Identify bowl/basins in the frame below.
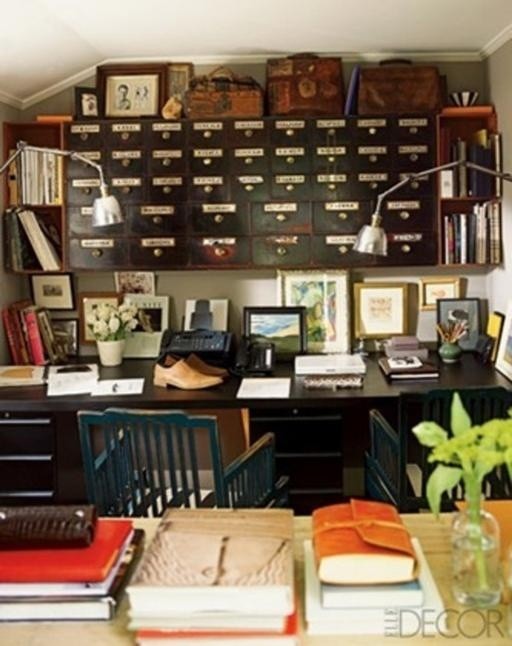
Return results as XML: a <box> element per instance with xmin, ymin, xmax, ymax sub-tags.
<box><xmin>450</xmin><ymin>90</ymin><xmax>479</xmax><ymax>107</ymax></box>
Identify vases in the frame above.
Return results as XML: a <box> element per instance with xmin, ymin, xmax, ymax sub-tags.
<box><xmin>96</xmin><ymin>338</ymin><xmax>125</xmax><ymax>368</ymax></box>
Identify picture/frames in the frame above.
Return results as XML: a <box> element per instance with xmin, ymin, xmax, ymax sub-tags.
<box><xmin>97</xmin><ymin>66</ymin><xmax>168</xmax><ymax>119</ymax></box>
<box><xmin>30</xmin><ymin>274</ymin><xmax>75</xmax><ymax>313</ymax></box>
<box><xmin>418</xmin><ymin>278</ymin><xmax>465</xmax><ymax>312</ymax></box>
<box><xmin>76</xmin><ymin>85</ymin><xmax>98</xmax><ymax>118</ymax></box>
<box><xmin>276</xmin><ymin>268</ymin><xmax>353</xmax><ymax>356</ymax></box>
<box><xmin>354</xmin><ymin>283</ymin><xmax>410</xmax><ymax>351</ymax></box>
<box><xmin>78</xmin><ymin>292</ymin><xmax>123</xmax><ymax>345</ymax></box>
<box><xmin>437</xmin><ymin>297</ymin><xmax>485</xmax><ymax>353</ymax></box>
<box><xmin>243</xmin><ymin>305</ymin><xmax>308</xmax><ymax>362</ymax></box>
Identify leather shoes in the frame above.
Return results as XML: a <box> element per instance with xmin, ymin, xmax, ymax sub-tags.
<box><xmin>151</xmin><ymin>357</ymin><xmax>226</xmax><ymax>391</ymax></box>
<box><xmin>163</xmin><ymin>351</ymin><xmax>230</xmax><ymax>379</ymax></box>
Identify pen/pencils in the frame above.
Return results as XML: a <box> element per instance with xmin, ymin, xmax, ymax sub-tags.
<box><xmin>57</xmin><ymin>366</ymin><xmax>91</xmax><ymax>373</ymax></box>
<box><xmin>435</xmin><ymin>319</ymin><xmax>470</xmax><ymax>344</ymax></box>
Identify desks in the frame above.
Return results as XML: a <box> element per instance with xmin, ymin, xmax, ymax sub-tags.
<box><xmin>0</xmin><ymin>341</ymin><xmax>511</xmax><ymax>516</ymax></box>
<box><xmin>0</xmin><ymin>506</ymin><xmax>512</xmax><ymax>646</ymax></box>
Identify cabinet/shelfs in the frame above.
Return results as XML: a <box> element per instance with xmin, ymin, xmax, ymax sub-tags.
<box><xmin>437</xmin><ymin>106</ymin><xmax>499</xmax><ymax>267</ymax></box>
<box><xmin>0</xmin><ymin>420</ymin><xmax>57</xmax><ymax>501</ymax></box>
<box><xmin>4</xmin><ymin>124</ymin><xmax>66</xmax><ymax>277</ymax></box>
<box><xmin>251</xmin><ymin>417</ymin><xmax>343</xmax><ymax>497</ymax></box>
<box><xmin>63</xmin><ymin>119</ymin><xmax>440</xmax><ymax>266</ymax></box>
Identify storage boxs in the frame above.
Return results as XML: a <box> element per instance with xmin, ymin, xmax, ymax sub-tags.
<box><xmin>349</xmin><ymin>57</ymin><xmax>448</xmax><ymax>117</ymax></box>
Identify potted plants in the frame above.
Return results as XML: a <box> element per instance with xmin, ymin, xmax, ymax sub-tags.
<box><xmin>435</xmin><ymin>319</ymin><xmax>470</xmax><ymax>363</ymax></box>
<box><xmin>411</xmin><ymin>392</ymin><xmax>512</xmax><ymax>613</ymax></box>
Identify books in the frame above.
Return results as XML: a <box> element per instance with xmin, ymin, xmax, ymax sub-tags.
<box><xmin>36</xmin><ymin>113</ymin><xmax>73</xmax><ymax>122</ymax></box>
<box><xmin>294</xmin><ymin>352</ymin><xmax>367</xmax><ymax>377</ymax></box>
<box><xmin>392</xmin><ymin>334</ymin><xmax>420</xmax><ymax>349</ymax></box>
<box><xmin>438</xmin><ymin>127</ymin><xmax>502</xmax><ymax>265</ymax></box>
<box><xmin>1</xmin><ymin>146</ymin><xmax>65</xmax><ymax>387</ymax></box>
<box><xmin>303</xmin><ymin>375</ymin><xmax>364</xmax><ymax>389</ymax></box>
<box><xmin>0</xmin><ymin>514</ymin><xmax>136</xmax><ymax>599</ymax></box>
<box><xmin>377</xmin><ymin>356</ymin><xmax>440</xmax><ymax>380</ymax></box>
<box><xmin>384</xmin><ymin>342</ymin><xmax>429</xmax><ymax>361</ymax></box>
<box><xmin>0</xmin><ymin>526</ymin><xmax>147</xmax><ymax>623</ymax></box>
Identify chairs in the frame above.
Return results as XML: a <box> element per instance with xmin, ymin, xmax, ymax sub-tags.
<box><xmin>72</xmin><ymin>408</ymin><xmax>293</xmax><ymax>517</ymax></box>
<box><xmin>368</xmin><ymin>385</ymin><xmax>512</xmax><ymax>510</ymax></box>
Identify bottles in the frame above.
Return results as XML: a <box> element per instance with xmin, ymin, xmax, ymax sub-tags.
<box><xmin>437</xmin><ymin>340</ymin><xmax>462</xmax><ymax>365</ymax></box>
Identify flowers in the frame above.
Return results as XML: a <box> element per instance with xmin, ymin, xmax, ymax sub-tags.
<box><xmin>87</xmin><ymin>300</ymin><xmax>139</xmax><ymax>342</ymax></box>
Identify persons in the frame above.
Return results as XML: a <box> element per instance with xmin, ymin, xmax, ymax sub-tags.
<box><xmin>82</xmin><ymin>96</ymin><xmax>97</xmax><ymax>117</ymax></box>
<box><xmin>114</xmin><ymin>82</ymin><xmax>133</xmax><ymax>110</ymax></box>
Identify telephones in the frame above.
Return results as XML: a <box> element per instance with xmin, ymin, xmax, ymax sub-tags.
<box><xmin>159</xmin><ymin>299</ymin><xmax>234</xmax><ymax>367</ymax></box>
<box><xmin>235</xmin><ymin>340</ymin><xmax>277</xmax><ymax>374</ymax></box>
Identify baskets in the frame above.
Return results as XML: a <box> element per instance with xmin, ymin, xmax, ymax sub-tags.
<box><xmin>361</xmin><ymin>56</ymin><xmax>444</xmax><ymax>117</ymax></box>
